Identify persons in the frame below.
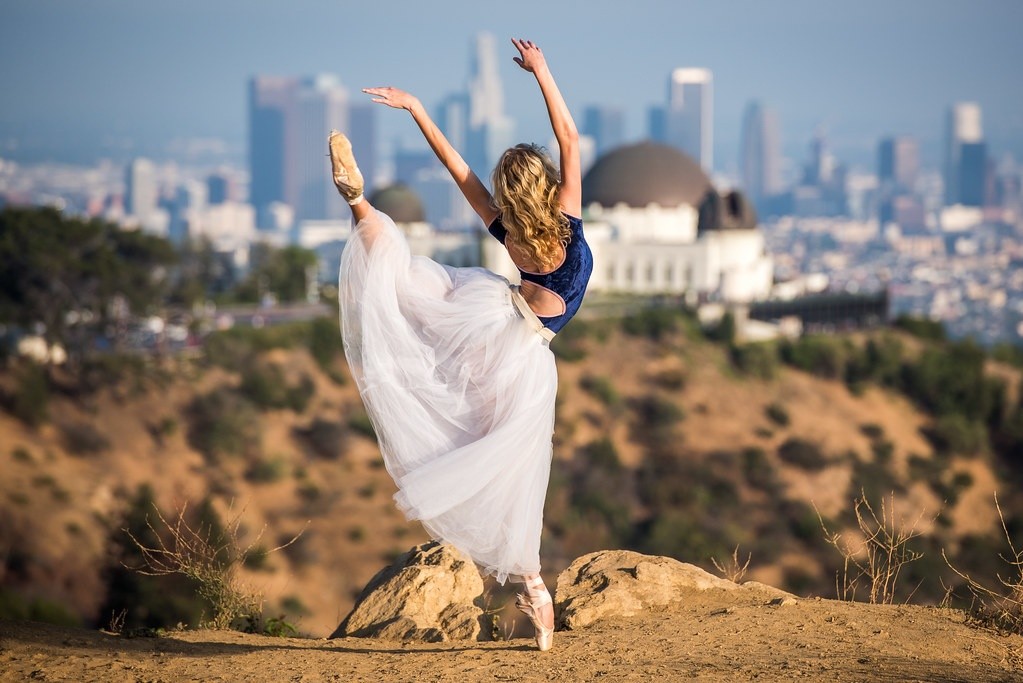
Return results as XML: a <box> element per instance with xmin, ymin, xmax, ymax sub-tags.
<box><xmin>327</xmin><ymin>36</ymin><xmax>593</xmax><ymax>651</ymax></box>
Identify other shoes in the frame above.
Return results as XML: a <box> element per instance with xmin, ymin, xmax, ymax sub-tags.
<box><xmin>515</xmin><ymin>591</ymin><xmax>556</xmax><ymax>652</ymax></box>
<box><xmin>327</xmin><ymin>132</ymin><xmax>364</xmax><ymax>199</ymax></box>
